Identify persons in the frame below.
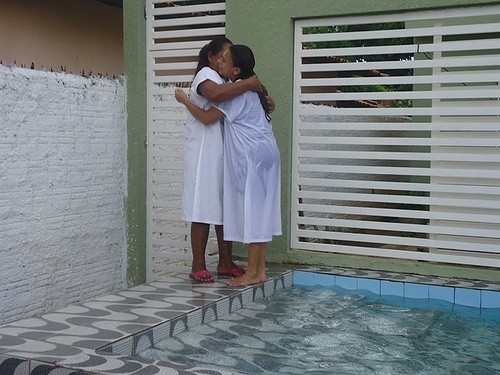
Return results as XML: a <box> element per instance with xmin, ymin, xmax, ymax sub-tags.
<box><xmin>176</xmin><ymin>44</ymin><xmax>282</xmax><ymax>286</ymax></box>
<box><xmin>179</xmin><ymin>38</ymin><xmax>274</xmax><ymax>283</ymax></box>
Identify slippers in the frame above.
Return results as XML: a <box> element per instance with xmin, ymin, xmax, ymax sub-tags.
<box><xmin>189</xmin><ymin>270</ymin><xmax>214</xmax><ymax>283</ymax></box>
<box><xmin>217</xmin><ymin>265</ymin><xmax>246</xmax><ymax>278</ymax></box>
